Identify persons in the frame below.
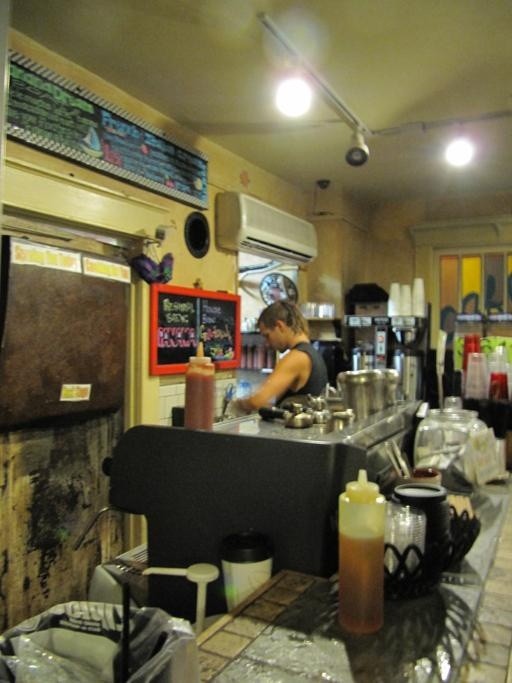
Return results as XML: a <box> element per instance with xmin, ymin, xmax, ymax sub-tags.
<box><xmin>233</xmin><ymin>299</ymin><xmax>330</xmax><ymax>414</ymax></box>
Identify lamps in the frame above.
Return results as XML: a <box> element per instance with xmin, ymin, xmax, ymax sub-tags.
<box><xmin>343</xmin><ymin>119</ymin><xmax>371</xmax><ymax>167</ymax></box>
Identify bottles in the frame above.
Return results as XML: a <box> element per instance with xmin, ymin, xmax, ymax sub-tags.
<box><xmin>413</xmin><ymin>395</ymin><xmax>489</xmax><ymax>492</ymax></box>
<box><xmin>185</xmin><ymin>340</ymin><xmax>216</xmax><ymax>430</ymax></box>
<box><xmin>338</xmin><ymin>468</ymin><xmax>387</xmax><ymax>634</ymax></box>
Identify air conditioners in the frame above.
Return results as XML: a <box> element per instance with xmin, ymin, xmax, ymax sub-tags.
<box><xmin>216</xmin><ymin>188</ymin><xmax>319</xmax><ymax>266</ymax></box>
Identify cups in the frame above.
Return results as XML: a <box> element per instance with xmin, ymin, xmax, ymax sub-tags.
<box><xmin>461</xmin><ymin>335</ymin><xmax>511</xmax><ymax>402</ymax></box>
<box><xmin>388</xmin><ymin>278</ymin><xmax>426</xmax><ymax>316</ymax></box>
<box><xmin>397</xmin><ymin>469</ymin><xmax>445</xmax><ymax>484</ymax></box>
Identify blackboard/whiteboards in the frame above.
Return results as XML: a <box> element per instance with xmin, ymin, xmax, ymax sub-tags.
<box><xmin>151</xmin><ymin>284</ymin><xmax>241</xmax><ymax>376</ymax></box>
